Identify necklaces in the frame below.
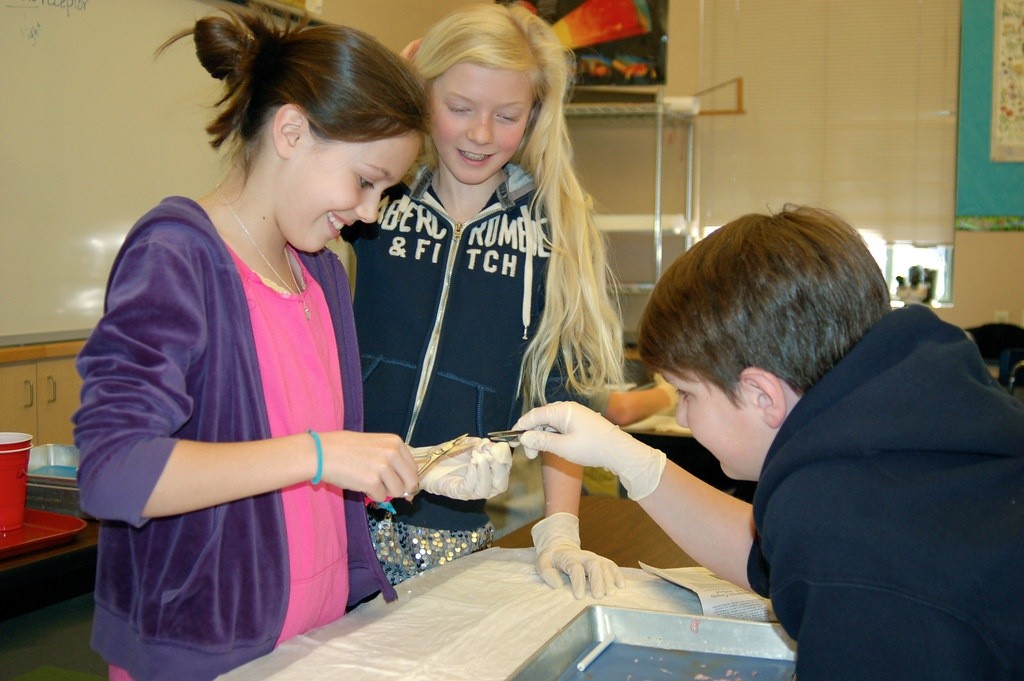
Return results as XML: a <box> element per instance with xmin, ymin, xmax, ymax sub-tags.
<box><xmin>212</xmin><ymin>186</ymin><xmax>311</xmax><ymax>320</ymax></box>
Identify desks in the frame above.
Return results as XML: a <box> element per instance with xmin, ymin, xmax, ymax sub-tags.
<box><xmin>0</xmin><ymin>516</ymin><xmax>100</xmax><ymax>621</ymax></box>
<box><xmin>215</xmin><ymin>495</ymin><xmax>780</xmax><ymax>681</ymax></box>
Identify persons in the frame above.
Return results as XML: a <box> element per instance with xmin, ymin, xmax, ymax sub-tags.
<box><xmin>334</xmin><ymin>3</ymin><xmax>624</xmax><ymax>600</ymax></box>
<box><xmin>70</xmin><ymin>5</ymin><xmax>513</xmax><ymax>681</ymax></box>
<box><xmin>508</xmin><ymin>203</ymin><xmax>1024</xmax><ymax>681</ymax></box>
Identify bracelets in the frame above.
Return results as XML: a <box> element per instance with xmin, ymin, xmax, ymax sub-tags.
<box><xmin>309</xmin><ymin>429</ymin><xmax>323</xmax><ymax>485</ymax></box>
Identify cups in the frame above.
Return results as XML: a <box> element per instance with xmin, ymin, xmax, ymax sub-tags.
<box><xmin>0</xmin><ymin>432</ymin><xmax>34</xmax><ymax>532</ymax></box>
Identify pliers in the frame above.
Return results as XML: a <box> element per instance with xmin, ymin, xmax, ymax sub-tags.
<box><xmin>410</xmin><ymin>432</ymin><xmax>471</xmax><ymax>478</ymax></box>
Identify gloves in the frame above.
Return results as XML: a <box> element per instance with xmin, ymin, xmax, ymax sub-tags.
<box><xmin>508</xmin><ymin>401</ymin><xmax>666</xmax><ymax>501</ymax></box>
<box><xmin>414</xmin><ymin>437</ymin><xmax>512</xmax><ymax>501</ymax></box>
<box><xmin>654</xmin><ymin>372</ymin><xmax>679</xmax><ymax>413</ymax></box>
<box><xmin>530</xmin><ymin>512</ymin><xmax>626</xmax><ymax>600</ymax></box>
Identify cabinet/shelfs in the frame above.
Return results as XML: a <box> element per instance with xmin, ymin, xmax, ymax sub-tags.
<box><xmin>0</xmin><ymin>327</ymin><xmax>94</xmax><ymax>444</ymax></box>
